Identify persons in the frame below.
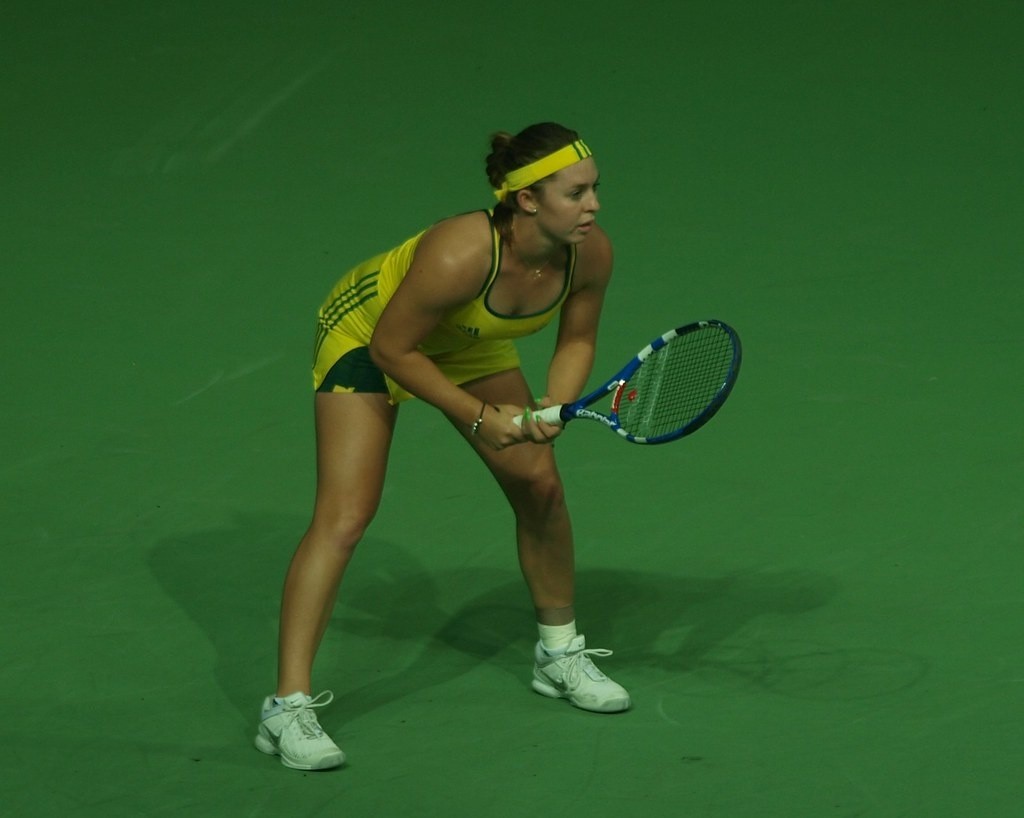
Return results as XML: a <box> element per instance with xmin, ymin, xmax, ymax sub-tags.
<box><xmin>254</xmin><ymin>121</ymin><xmax>631</xmax><ymax>773</ymax></box>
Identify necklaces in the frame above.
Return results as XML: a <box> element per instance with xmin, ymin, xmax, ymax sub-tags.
<box><xmin>507</xmin><ymin>235</ymin><xmax>561</xmax><ymax>281</ymax></box>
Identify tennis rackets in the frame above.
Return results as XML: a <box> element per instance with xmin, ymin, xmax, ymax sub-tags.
<box><xmin>512</xmin><ymin>320</ymin><xmax>744</xmax><ymax>445</ymax></box>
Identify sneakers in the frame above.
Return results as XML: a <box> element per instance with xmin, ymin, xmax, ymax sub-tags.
<box><xmin>254</xmin><ymin>691</ymin><xmax>346</xmax><ymax>772</ymax></box>
<box><xmin>531</xmin><ymin>634</ymin><xmax>631</xmax><ymax>712</ymax></box>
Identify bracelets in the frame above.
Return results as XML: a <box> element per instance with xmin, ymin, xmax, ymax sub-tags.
<box><xmin>472</xmin><ymin>397</ymin><xmax>500</xmax><ymax>436</ymax></box>
